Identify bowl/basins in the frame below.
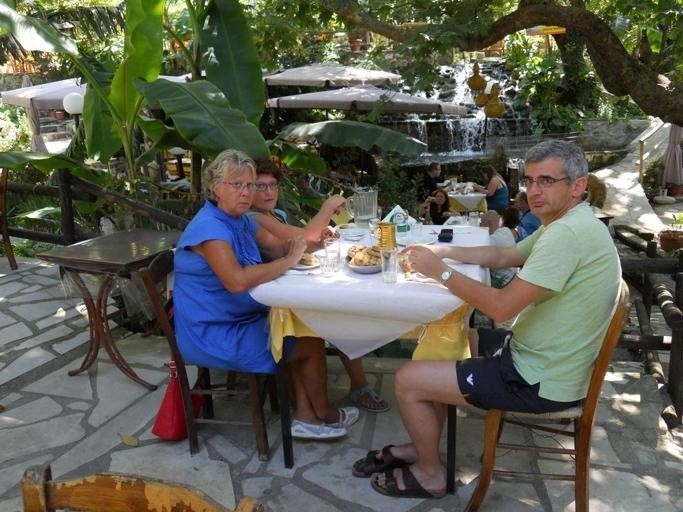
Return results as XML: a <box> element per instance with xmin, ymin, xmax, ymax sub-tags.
<box><xmin>344</xmin><ymin>255</ymin><xmax>389</xmax><ymax>274</ymax></box>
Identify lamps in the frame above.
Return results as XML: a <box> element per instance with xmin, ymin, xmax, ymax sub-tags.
<box><xmin>62</xmin><ymin>93</ymin><xmax>84</xmax><ymax>132</ymax></box>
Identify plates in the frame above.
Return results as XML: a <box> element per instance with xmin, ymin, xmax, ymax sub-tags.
<box><xmin>292</xmin><ymin>258</ymin><xmax>321</xmax><ymax>270</ymax></box>
<box><xmin>396</xmin><ymin>234</ymin><xmax>438</xmax><ymax>247</ymax></box>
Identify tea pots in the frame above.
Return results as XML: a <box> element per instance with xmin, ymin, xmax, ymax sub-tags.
<box><xmin>346</xmin><ymin>189</ymin><xmax>378</xmax><ymax>229</ymax></box>
<box><xmin>469</xmin><ymin>212</ymin><xmax>479</xmax><ymax>227</ymax></box>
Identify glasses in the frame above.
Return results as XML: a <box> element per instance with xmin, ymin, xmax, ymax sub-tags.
<box><xmin>216</xmin><ymin>180</ymin><xmax>256</xmax><ymax>190</ymax></box>
<box><xmin>522</xmin><ymin>175</ymin><xmax>570</xmax><ymax>186</ymax></box>
<box><xmin>254</xmin><ymin>179</ymin><xmax>281</xmax><ymax>192</ymax></box>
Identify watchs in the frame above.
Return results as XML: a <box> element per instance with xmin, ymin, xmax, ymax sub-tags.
<box><xmin>438</xmin><ymin>266</ymin><xmax>455</xmax><ymax>285</ymax></box>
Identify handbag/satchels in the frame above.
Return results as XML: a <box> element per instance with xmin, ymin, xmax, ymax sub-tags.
<box><xmin>153</xmin><ymin>374</ymin><xmax>203</xmax><ymax>441</ymax></box>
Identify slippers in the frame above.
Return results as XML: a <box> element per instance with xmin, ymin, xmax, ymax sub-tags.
<box><xmin>352</xmin><ymin>445</ymin><xmax>413</xmax><ymax>478</ymax></box>
<box><xmin>346</xmin><ymin>384</ymin><xmax>388</xmax><ymax>413</ymax></box>
<box><xmin>370</xmin><ymin>466</ymin><xmax>446</xmax><ymax>499</ymax></box>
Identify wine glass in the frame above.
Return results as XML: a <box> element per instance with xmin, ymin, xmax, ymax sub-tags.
<box><xmin>451</xmin><ymin>178</ymin><xmax>457</xmax><ymax>192</ymax></box>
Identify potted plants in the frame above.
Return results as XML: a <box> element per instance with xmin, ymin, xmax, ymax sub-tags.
<box><xmin>655</xmin><ymin>216</ymin><xmax>682</xmax><ymax>252</ymax></box>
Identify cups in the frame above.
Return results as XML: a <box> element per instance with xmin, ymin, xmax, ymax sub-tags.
<box><xmin>379</xmin><ymin>249</ymin><xmax>398</xmax><ymax>283</ymax></box>
<box><xmin>324</xmin><ymin>238</ymin><xmax>340</xmax><ymax>272</ymax></box>
<box><xmin>377</xmin><ymin>207</ymin><xmax>382</xmax><ymax>221</ymax></box>
<box><xmin>410</xmin><ymin>224</ymin><xmax>424</xmax><ymax>243</ymax></box>
<box><xmin>394</xmin><ymin>209</ymin><xmax>408</xmax><ymax>241</ymax></box>
<box><xmin>318</xmin><ymin>256</ymin><xmax>337</xmax><ymax>277</ymax></box>
<box><xmin>368</xmin><ymin>218</ymin><xmax>380</xmax><ymax>239</ymax></box>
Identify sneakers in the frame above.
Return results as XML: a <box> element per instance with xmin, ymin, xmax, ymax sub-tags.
<box><xmin>290</xmin><ymin>418</ymin><xmax>347</xmax><ymax>439</ymax></box>
<box><xmin>326</xmin><ymin>407</ymin><xmax>360</xmax><ymax>428</ymax></box>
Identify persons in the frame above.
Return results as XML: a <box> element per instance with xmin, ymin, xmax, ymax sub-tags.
<box><xmin>478</xmin><ymin>211</ymin><xmax>518</xmax><ymax>289</ymax></box>
<box><xmin>416</xmin><ymin>163</ymin><xmax>454</xmax><ymax>202</ymax></box>
<box><xmin>373</xmin><ymin>139</ymin><xmax>623</xmax><ymax>493</ymax></box>
<box><xmin>501</xmin><ymin>207</ymin><xmax>524</xmax><ymax>243</ymax></box>
<box><xmin>245</xmin><ymin>155</ymin><xmax>390</xmax><ymax>411</ymax></box>
<box><xmin>472</xmin><ymin>164</ymin><xmax>512</xmax><ymax>211</ymax></box>
<box><xmin>173</xmin><ymin>147</ymin><xmax>360</xmax><ymax>442</ymax></box>
<box><xmin>514</xmin><ymin>191</ymin><xmax>541</xmax><ymax>235</ymax></box>
<box><xmin>420</xmin><ymin>189</ymin><xmax>462</xmax><ymax>223</ymax></box>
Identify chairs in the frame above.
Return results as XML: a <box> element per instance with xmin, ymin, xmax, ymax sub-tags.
<box><xmin>20</xmin><ymin>462</ymin><xmax>267</xmax><ymax>512</ymax></box>
<box><xmin>143</xmin><ymin>247</ymin><xmax>277</xmax><ymax>460</ymax></box>
<box><xmin>464</xmin><ymin>279</ymin><xmax>626</xmax><ymax>512</ymax></box>
